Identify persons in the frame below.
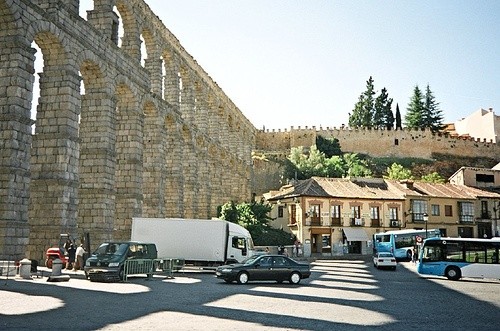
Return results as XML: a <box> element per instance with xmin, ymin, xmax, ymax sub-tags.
<box><xmin>277</xmin><ymin>242</ymin><xmax>303</xmax><ymax>258</ymax></box>
<box><xmin>474</xmin><ymin>252</ymin><xmax>497</xmax><ymax>264</ymax></box>
<box><xmin>265</xmin><ymin>247</ymin><xmax>270</xmax><ymax>252</ymax></box>
<box><xmin>410</xmin><ymin>248</ymin><xmax>417</xmax><ymax>263</ymax></box>
<box><xmin>62</xmin><ymin>238</ymin><xmax>89</xmax><ymax>272</ymax></box>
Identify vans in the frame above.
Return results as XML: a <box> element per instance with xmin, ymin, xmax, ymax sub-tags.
<box><xmin>84</xmin><ymin>240</ymin><xmax>159</xmax><ymax>280</ymax></box>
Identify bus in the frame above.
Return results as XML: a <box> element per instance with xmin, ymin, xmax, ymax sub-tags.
<box><xmin>374</xmin><ymin>228</ymin><xmax>441</xmax><ymax>262</ymax></box>
<box><xmin>417</xmin><ymin>237</ymin><xmax>500</xmax><ymax>282</ymax></box>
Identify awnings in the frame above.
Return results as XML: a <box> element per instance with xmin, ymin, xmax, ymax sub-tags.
<box><xmin>343</xmin><ymin>227</ymin><xmax>369</xmax><ymax>242</ymax></box>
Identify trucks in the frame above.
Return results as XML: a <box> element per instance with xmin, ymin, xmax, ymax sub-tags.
<box><xmin>131</xmin><ymin>217</ymin><xmax>255</xmax><ymax>270</ymax></box>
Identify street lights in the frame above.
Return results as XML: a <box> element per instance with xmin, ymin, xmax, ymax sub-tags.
<box><xmin>423</xmin><ymin>212</ymin><xmax>429</xmax><ymax>239</ymax></box>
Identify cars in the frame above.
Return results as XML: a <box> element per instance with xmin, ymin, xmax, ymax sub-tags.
<box><xmin>215</xmin><ymin>255</ymin><xmax>311</xmax><ymax>286</ymax></box>
<box><xmin>372</xmin><ymin>251</ymin><xmax>397</xmax><ymax>271</ymax></box>
